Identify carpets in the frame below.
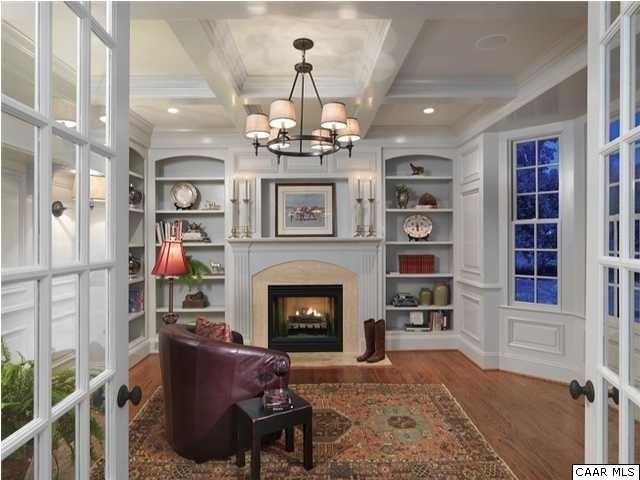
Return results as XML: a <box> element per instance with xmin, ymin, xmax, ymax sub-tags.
<box><xmin>73</xmin><ymin>380</ymin><xmax>519</xmax><ymax>480</ymax></box>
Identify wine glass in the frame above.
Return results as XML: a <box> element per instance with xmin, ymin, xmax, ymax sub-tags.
<box><xmin>273</xmin><ymin>359</ymin><xmax>290</xmax><ymax>392</ymax></box>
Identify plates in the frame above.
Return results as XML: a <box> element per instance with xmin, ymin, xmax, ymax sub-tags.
<box><xmin>170</xmin><ymin>182</ymin><xmax>197</xmax><ymax>208</ymax></box>
<box><xmin>403</xmin><ymin>215</ymin><xmax>432</xmax><ymax>238</ymax></box>
<box><xmin>129</xmin><ymin>255</ymin><xmax>137</xmax><ymax>280</ymax></box>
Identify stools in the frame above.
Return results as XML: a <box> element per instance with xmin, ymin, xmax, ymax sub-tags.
<box><xmin>232</xmin><ymin>391</ymin><xmax>313</xmax><ymax>480</ymax></box>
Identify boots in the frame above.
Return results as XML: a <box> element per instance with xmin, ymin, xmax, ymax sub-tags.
<box><xmin>356</xmin><ymin>319</ymin><xmax>375</xmax><ymax>361</ymax></box>
<box><xmin>367</xmin><ymin>319</ymin><xmax>386</xmax><ymax>361</ymax></box>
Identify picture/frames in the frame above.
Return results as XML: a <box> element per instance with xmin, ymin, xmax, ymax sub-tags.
<box><xmin>273</xmin><ymin>182</ymin><xmax>337</xmax><ymax>238</ymax></box>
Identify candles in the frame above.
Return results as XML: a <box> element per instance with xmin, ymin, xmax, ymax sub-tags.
<box><xmin>357</xmin><ymin>175</ymin><xmax>362</xmax><ymax>198</ymax></box>
<box><xmin>244</xmin><ymin>177</ymin><xmax>250</xmax><ymax>198</ymax></box>
<box><xmin>231</xmin><ymin>177</ymin><xmax>237</xmax><ymax>199</ymax></box>
<box><xmin>368</xmin><ymin>176</ymin><xmax>374</xmax><ymax>197</ymax></box>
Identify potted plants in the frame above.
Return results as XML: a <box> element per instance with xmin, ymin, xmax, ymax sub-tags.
<box><xmin>1</xmin><ymin>337</ymin><xmax>105</xmax><ymax>480</ymax></box>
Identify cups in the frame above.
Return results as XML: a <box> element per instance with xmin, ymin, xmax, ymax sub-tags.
<box><xmin>133</xmin><ymin>191</ymin><xmax>142</xmax><ymax>203</ymax></box>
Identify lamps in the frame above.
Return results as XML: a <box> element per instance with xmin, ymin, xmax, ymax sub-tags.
<box><xmin>241</xmin><ymin>36</ymin><xmax>363</xmax><ymax>167</ymax></box>
<box><xmin>51</xmin><ymin>169</ymin><xmax>77</xmax><ymax>199</ymax></box>
<box><xmin>52</xmin><ymin>175</ymin><xmax>107</xmax><ymax>218</ymax></box>
<box><xmin>149</xmin><ymin>240</ymin><xmax>192</xmax><ymax>327</ymax></box>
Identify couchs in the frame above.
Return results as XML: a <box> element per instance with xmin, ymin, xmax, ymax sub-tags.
<box><xmin>156</xmin><ymin>317</ymin><xmax>291</xmax><ymax>463</ymax></box>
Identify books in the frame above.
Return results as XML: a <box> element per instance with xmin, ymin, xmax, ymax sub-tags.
<box><xmin>397</xmin><ymin>253</ymin><xmax>437</xmax><ymax>273</ymax></box>
<box><xmin>428</xmin><ymin>311</ymin><xmax>448</xmax><ymax>332</ymax></box>
<box><xmin>156</xmin><ymin>217</ymin><xmax>211</xmax><ymax>245</ymax></box>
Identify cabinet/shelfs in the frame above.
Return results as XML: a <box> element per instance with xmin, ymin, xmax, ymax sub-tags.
<box><xmin>153</xmin><ymin>175</ymin><xmax>227</xmax><ymax>337</ymax></box>
<box><xmin>384</xmin><ymin>174</ymin><xmax>456</xmax><ymax>335</ymax></box>
<box><xmin>127</xmin><ymin>169</ymin><xmax>146</xmax><ymax>348</ymax></box>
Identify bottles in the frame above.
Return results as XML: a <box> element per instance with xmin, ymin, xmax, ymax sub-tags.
<box><xmin>129</xmin><ymin>183</ymin><xmax>135</xmax><ymax>206</ymax></box>
<box><xmin>171</xmin><ymin>221</ymin><xmax>176</xmax><ymax>235</ymax></box>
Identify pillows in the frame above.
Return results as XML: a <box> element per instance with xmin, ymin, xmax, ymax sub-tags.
<box><xmin>195</xmin><ymin>317</ymin><xmax>232</xmax><ymax>345</ymax></box>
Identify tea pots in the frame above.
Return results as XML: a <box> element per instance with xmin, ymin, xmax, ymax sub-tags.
<box><xmin>188</xmin><ymin>222</ymin><xmax>202</xmax><ymax>229</ymax></box>
